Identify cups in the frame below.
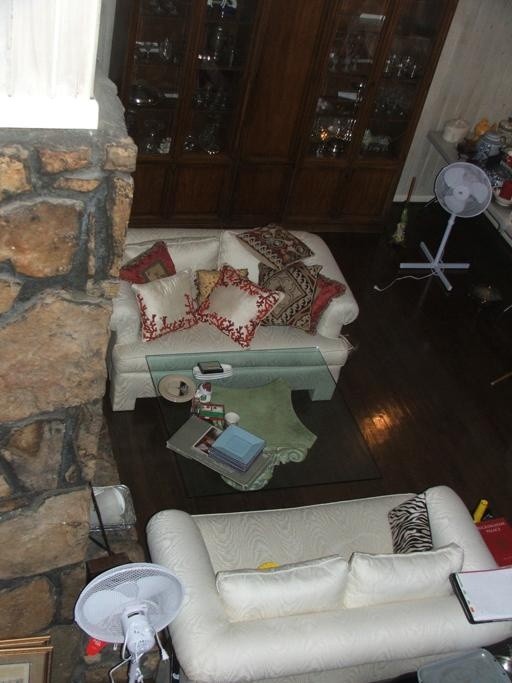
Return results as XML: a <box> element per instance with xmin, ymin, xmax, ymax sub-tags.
<box><xmin>223</xmin><ymin>411</ymin><xmax>241</xmax><ymax>429</ymax></box>
<box><xmin>500</xmin><ymin>178</ymin><xmax>512</xmax><ymax>198</ymax></box>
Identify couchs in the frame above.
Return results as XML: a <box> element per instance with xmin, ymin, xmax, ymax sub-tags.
<box><xmin>107</xmin><ymin>228</ymin><xmax>360</xmax><ymax>412</ymax></box>
<box><xmin>145</xmin><ymin>484</ymin><xmax>511</xmax><ymax>682</ymax></box>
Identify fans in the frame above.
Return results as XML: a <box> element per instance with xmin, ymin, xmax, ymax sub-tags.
<box><xmin>74</xmin><ymin>562</ymin><xmax>183</xmax><ymax>683</ymax></box>
<box><xmin>399</xmin><ymin>162</ymin><xmax>493</xmax><ymax>291</ymax></box>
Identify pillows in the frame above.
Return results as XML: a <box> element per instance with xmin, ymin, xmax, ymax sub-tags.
<box><xmin>120</xmin><ymin>223</ymin><xmax>349</xmax><ymax>350</ymax></box>
<box><xmin>218</xmin><ymin>494</ymin><xmax>462</xmax><ymax>617</ymax></box>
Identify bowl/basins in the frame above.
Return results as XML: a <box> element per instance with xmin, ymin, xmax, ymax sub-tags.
<box><xmin>493</xmin><ymin>189</ymin><xmax>512</xmax><ymax>206</ymax></box>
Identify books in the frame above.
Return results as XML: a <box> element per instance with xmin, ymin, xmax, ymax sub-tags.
<box><xmin>357</xmin><ymin>4</ymin><xmax>386</xmax><ymax>19</ymax></box>
<box><xmin>165</xmin><ymin>413</ymin><xmax>272</xmax><ymax>489</ymax></box>
<box><xmin>448</xmin><ymin>564</ymin><xmax>512</xmax><ymax>624</ymax></box>
<box><xmin>331</xmin><ymin>76</ymin><xmax>362</xmax><ymax>100</ymax></box>
<box><xmin>454</xmin><ymin>566</ymin><xmax>512</xmax><ymax>622</ymax></box>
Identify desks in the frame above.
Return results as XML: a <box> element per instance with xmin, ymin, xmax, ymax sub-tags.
<box><xmin>427</xmin><ymin>121</ymin><xmax>512</xmax><ymax>249</ymax></box>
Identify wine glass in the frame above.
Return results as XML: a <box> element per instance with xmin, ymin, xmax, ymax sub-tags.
<box><xmin>158</xmin><ymin>37</ymin><xmax>174</xmax><ymax>64</ymax></box>
<box><xmin>142</xmin><ymin>39</ymin><xmax>155</xmax><ymax>63</ymax></box>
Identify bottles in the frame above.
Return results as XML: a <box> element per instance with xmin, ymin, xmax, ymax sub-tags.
<box><xmin>471</xmin><ymin>115</ymin><xmax>512</xmax><ymax>160</ymax></box>
<box><xmin>442</xmin><ymin>117</ymin><xmax>469</xmax><ymax>142</ymax></box>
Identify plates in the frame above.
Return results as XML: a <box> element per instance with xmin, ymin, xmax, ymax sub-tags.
<box><xmin>208</xmin><ymin>423</ymin><xmax>267</xmax><ymax>472</ymax></box>
<box><xmin>157</xmin><ymin>372</ymin><xmax>197</xmax><ymax>404</ymax></box>
